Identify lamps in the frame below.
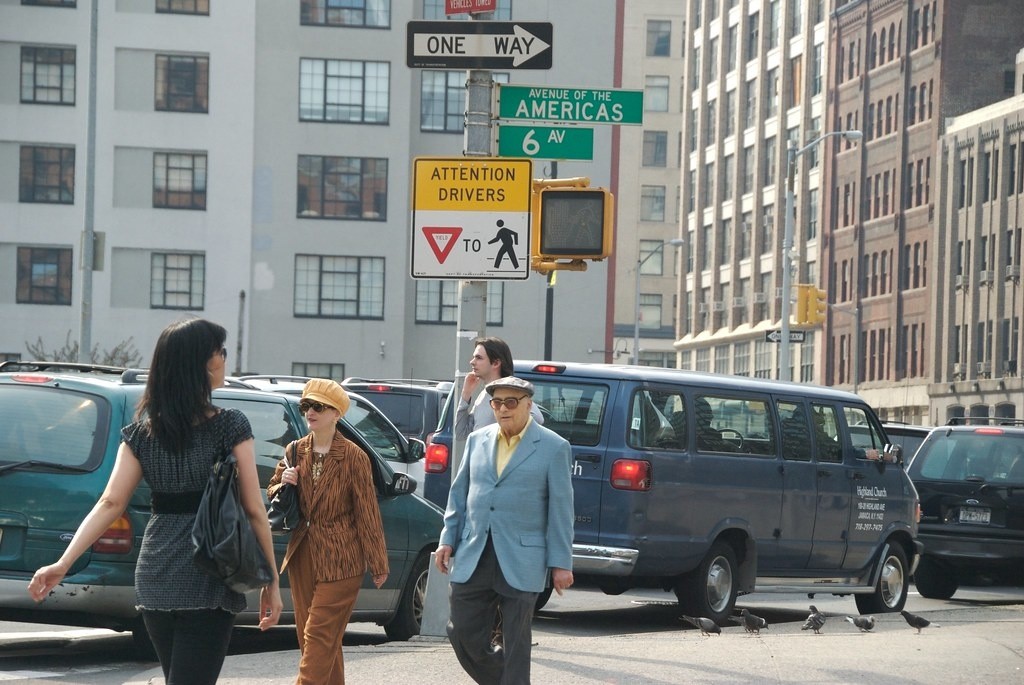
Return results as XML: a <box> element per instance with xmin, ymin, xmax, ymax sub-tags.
<box><xmin>971</xmin><ymin>381</ymin><xmax>979</xmax><ymax>392</ymax></box>
<box><xmin>997</xmin><ymin>380</ymin><xmax>1004</xmax><ymax>391</ymax></box>
<box><xmin>948</xmin><ymin>383</ymin><xmax>955</xmax><ymax>394</ymax></box>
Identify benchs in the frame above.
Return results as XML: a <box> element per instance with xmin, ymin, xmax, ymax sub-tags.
<box><xmin>541</xmin><ymin>419</ymin><xmax>636</xmax><ymax>448</ymax></box>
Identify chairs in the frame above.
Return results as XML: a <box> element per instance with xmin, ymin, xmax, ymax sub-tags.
<box><xmin>967</xmin><ymin>456</ymin><xmax>993</xmax><ymax>475</ymax></box>
<box><xmin>780</xmin><ymin>419</ymin><xmax>815</xmax><ymax>460</ymax></box>
<box><xmin>662</xmin><ymin>411</ymin><xmax>686</xmax><ymax>448</ymax></box>
<box><xmin>1007</xmin><ymin>452</ymin><xmax>1024</xmax><ymax>483</ymax></box>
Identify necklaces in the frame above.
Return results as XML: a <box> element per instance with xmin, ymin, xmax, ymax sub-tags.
<box><xmin>312</xmin><ymin>433</ymin><xmax>331</xmax><ymax>457</ymax></box>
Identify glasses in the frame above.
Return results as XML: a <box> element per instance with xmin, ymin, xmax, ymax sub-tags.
<box><xmin>489</xmin><ymin>395</ymin><xmax>529</xmax><ymax>410</ymax></box>
<box><xmin>299</xmin><ymin>401</ymin><xmax>337</xmax><ymax>412</ymax></box>
<box><xmin>207</xmin><ymin>347</ymin><xmax>228</xmax><ymax>363</ymax></box>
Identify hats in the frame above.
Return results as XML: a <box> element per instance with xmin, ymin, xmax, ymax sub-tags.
<box><xmin>486</xmin><ymin>376</ymin><xmax>534</xmax><ymax>397</ymax></box>
<box><xmin>300</xmin><ymin>378</ymin><xmax>350</xmax><ymax>417</ymax></box>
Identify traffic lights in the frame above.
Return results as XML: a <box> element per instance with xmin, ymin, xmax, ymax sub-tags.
<box><xmin>806</xmin><ymin>285</ymin><xmax>827</xmax><ymax>325</ymax></box>
<box><xmin>537</xmin><ymin>186</ymin><xmax>613</xmax><ymax>260</ymax></box>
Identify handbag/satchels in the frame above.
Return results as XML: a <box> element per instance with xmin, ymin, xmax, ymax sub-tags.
<box><xmin>266</xmin><ymin>440</ymin><xmax>301</xmax><ymax>533</ymax></box>
<box><xmin>193</xmin><ymin>409</ymin><xmax>273</xmax><ymax>593</ymax></box>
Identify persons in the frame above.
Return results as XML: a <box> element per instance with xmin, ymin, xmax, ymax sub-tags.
<box><xmin>676</xmin><ymin>396</ymin><xmax>744</xmax><ymax>453</ymax></box>
<box><xmin>267</xmin><ymin>378</ymin><xmax>390</xmax><ymax>685</ymax></box>
<box><xmin>455</xmin><ymin>337</ymin><xmax>544</xmax><ymax>652</ymax></box>
<box><xmin>435</xmin><ymin>375</ymin><xmax>573</xmax><ymax>685</ymax></box>
<box><xmin>28</xmin><ymin>315</ymin><xmax>283</xmax><ymax>685</ymax></box>
<box><xmin>770</xmin><ymin>404</ymin><xmax>880</xmax><ymax>460</ymax></box>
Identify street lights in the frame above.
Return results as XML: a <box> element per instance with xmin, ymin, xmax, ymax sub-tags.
<box><xmin>632</xmin><ymin>239</ymin><xmax>685</xmax><ymax>365</ymax></box>
<box><xmin>779</xmin><ymin>130</ymin><xmax>863</xmax><ymax>381</ymax></box>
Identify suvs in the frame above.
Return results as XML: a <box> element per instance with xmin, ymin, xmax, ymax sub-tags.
<box><xmin>340</xmin><ymin>376</ymin><xmax>454</xmax><ymax>444</ymax></box>
<box><xmin>0</xmin><ymin>360</ymin><xmax>446</xmax><ymax>660</ymax></box>
<box><xmin>224</xmin><ymin>375</ymin><xmax>426</xmax><ymax>498</ymax></box>
<box><xmin>833</xmin><ymin>416</ymin><xmax>1024</xmax><ymax>600</ymax></box>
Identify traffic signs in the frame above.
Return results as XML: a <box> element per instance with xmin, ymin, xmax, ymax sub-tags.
<box><xmin>766</xmin><ymin>331</ymin><xmax>806</xmax><ymax>342</ymax></box>
<box><xmin>406</xmin><ymin>21</ymin><xmax>552</xmax><ymax>70</ymax></box>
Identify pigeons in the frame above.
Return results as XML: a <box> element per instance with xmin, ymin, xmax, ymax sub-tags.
<box><xmin>679</xmin><ymin>616</ymin><xmax>722</xmax><ymax>636</ymax></box>
<box><xmin>728</xmin><ymin>609</ymin><xmax>770</xmax><ymax>636</ymax></box>
<box><xmin>900</xmin><ymin>610</ymin><xmax>941</xmax><ymax>636</ymax></box>
<box><xmin>845</xmin><ymin>616</ymin><xmax>877</xmax><ymax>633</ymax></box>
<box><xmin>801</xmin><ymin>605</ymin><xmax>826</xmax><ymax>635</ymax></box>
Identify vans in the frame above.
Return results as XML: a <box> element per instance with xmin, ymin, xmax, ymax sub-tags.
<box><xmin>423</xmin><ymin>359</ymin><xmax>925</xmax><ymax>626</ymax></box>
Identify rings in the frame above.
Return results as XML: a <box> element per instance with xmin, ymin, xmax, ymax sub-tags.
<box><xmin>286</xmin><ymin>474</ymin><xmax>288</xmax><ymax>479</ymax></box>
